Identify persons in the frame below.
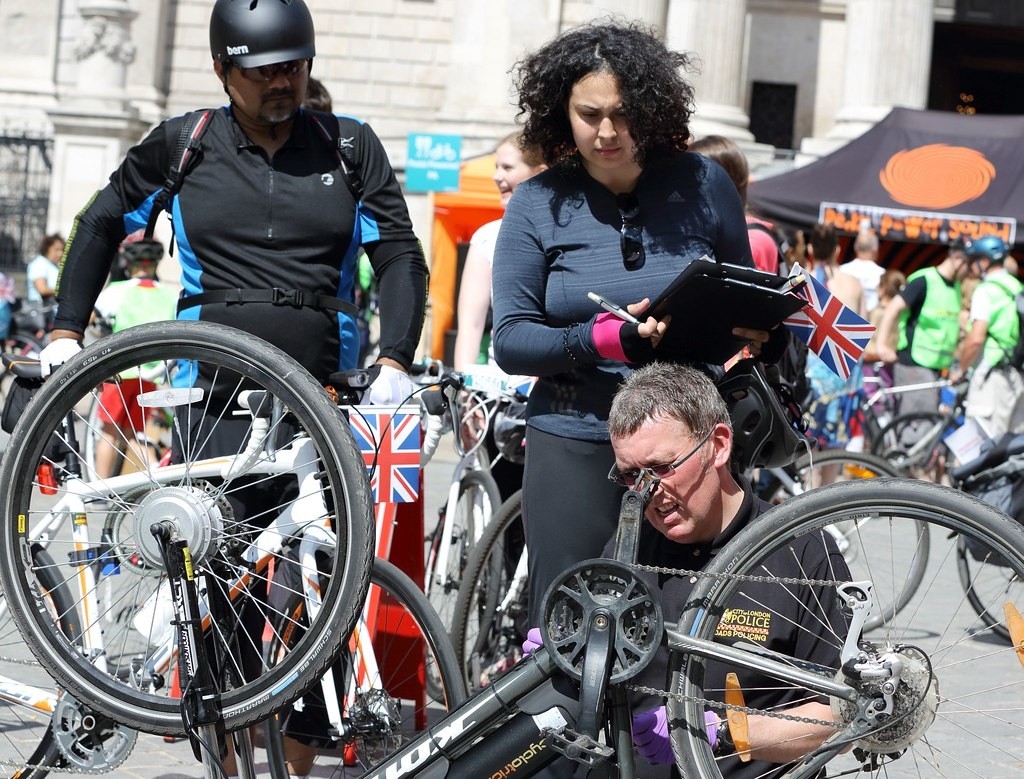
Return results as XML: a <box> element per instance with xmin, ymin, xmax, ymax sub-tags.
<box><xmin>520</xmin><ymin>361</ymin><xmax>863</xmax><ymax>779</ymax></box>
<box><xmin>0</xmin><ymin>77</ymin><xmax>383</xmax><ymax>512</ymax></box>
<box><xmin>448</xmin><ymin>130</ymin><xmax>547</xmax><ymax>686</ymax></box>
<box><xmin>490</xmin><ymin>18</ymin><xmax>777</xmax><ymax>616</ymax></box>
<box><xmin>688</xmin><ymin>135</ymin><xmax>1024</xmax><ymax>502</ymax></box>
<box><xmin>39</xmin><ymin>0</ymin><xmax>435</xmax><ymax>779</ymax></box>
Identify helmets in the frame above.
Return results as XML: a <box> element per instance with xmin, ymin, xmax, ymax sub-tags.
<box><xmin>209</xmin><ymin>1</ymin><xmax>315</xmax><ymax>69</ymax></box>
<box><xmin>949</xmin><ymin>234</ymin><xmax>975</xmax><ymax>249</ymax></box>
<box><xmin>118</xmin><ymin>228</ymin><xmax>163</xmax><ymax>261</ymax></box>
<box><xmin>715</xmin><ymin>359</ymin><xmax>818</xmax><ymax>468</ymax></box>
<box><xmin>965</xmin><ymin>235</ymin><xmax>1007</xmax><ymax>262</ymax></box>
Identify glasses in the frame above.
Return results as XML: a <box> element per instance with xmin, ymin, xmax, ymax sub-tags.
<box><xmin>227</xmin><ymin>58</ymin><xmax>309</xmax><ymax>82</ymax></box>
<box><xmin>615</xmin><ymin>191</ymin><xmax>645</xmax><ymax>271</ymax></box>
<box><xmin>607</xmin><ymin>415</ymin><xmax>719</xmax><ymax>486</ymax></box>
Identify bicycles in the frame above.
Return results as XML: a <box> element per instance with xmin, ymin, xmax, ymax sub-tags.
<box><xmin>0</xmin><ymin>318</ymin><xmax>1023</xmax><ymax>779</ymax></box>
<box><xmin>0</xmin><ymin>309</ymin><xmax>1023</xmax><ymax>779</ymax></box>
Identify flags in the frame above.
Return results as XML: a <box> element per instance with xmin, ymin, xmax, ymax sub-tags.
<box><xmin>348</xmin><ymin>405</ymin><xmax>419</xmax><ymax>504</ymax></box>
<box><xmin>783</xmin><ymin>260</ymin><xmax>877</xmax><ymax>382</ymax></box>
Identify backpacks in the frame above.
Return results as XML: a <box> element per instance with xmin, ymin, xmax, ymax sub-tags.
<box><xmin>985</xmin><ymin>275</ymin><xmax>1024</xmax><ymax>369</ymax></box>
<box><xmin>747</xmin><ymin>223</ymin><xmax>809</xmax><ymax>405</ymax></box>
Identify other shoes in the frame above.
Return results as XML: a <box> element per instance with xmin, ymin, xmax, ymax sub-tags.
<box><xmin>84</xmin><ymin>496</ymin><xmax>106</xmax><ymax>502</ymax></box>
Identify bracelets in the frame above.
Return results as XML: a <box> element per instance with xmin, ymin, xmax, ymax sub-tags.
<box><xmin>561</xmin><ymin>321</ymin><xmax>586</xmax><ymax>361</ymax></box>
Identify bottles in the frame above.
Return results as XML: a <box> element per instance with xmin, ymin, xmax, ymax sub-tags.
<box><xmin>133</xmin><ymin>567</ymin><xmax>206</xmax><ymax>643</ymax></box>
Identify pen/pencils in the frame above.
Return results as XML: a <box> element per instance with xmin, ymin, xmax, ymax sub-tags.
<box><xmin>778</xmin><ymin>274</ymin><xmax>806</xmax><ymax>294</ymax></box>
<box><xmin>587</xmin><ymin>292</ymin><xmax>660</xmax><ymax>338</ymax></box>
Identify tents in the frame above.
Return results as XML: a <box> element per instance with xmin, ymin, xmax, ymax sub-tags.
<box><xmin>747</xmin><ymin>105</ymin><xmax>1024</xmax><ymax>278</ymax></box>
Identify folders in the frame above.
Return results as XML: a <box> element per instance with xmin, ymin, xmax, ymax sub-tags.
<box><xmin>636</xmin><ymin>259</ymin><xmax>809</xmax><ymax>367</ymax></box>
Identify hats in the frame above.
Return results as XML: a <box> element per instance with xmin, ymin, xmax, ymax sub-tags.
<box><xmin>780</xmin><ymin>228</ymin><xmax>799</xmax><ymax>253</ymax></box>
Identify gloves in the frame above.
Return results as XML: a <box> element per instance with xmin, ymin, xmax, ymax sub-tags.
<box><xmin>358</xmin><ymin>365</ymin><xmax>421</xmax><ymax>406</ymax></box>
<box><xmin>38</xmin><ymin>338</ymin><xmax>84</xmax><ymax>379</ymax></box>
<box><xmin>631</xmin><ymin>704</ymin><xmax>725</xmax><ymax>765</ymax></box>
<box><xmin>522</xmin><ymin>627</ymin><xmax>543</xmax><ymax>659</ymax></box>
